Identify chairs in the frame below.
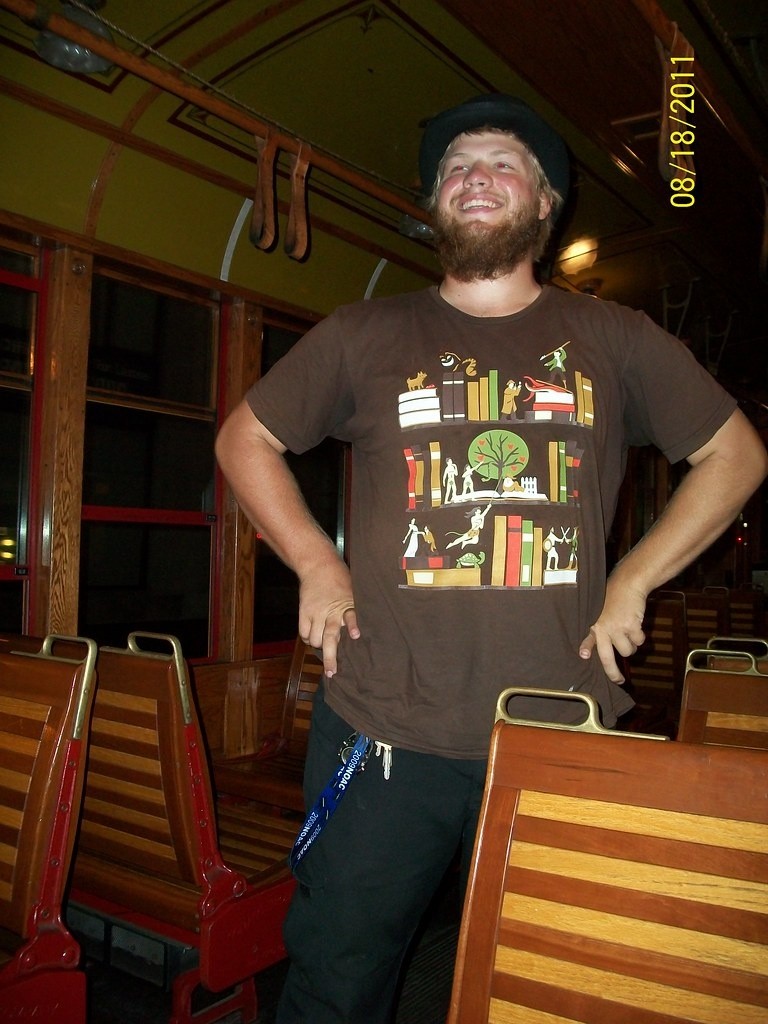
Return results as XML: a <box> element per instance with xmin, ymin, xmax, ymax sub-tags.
<box><xmin>1</xmin><ymin>575</ymin><xmax>766</xmax><ymax>1024</ymax></box>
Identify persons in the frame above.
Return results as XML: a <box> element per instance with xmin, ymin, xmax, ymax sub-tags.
<box><xmin>216</xmin><ymin>93</ymin><xmax>764</xmax><ymax>1024</ymax></box>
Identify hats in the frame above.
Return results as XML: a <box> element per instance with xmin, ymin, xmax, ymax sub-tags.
<box><xmin>417</xmin><ymin>92</ymin><xmax>573</xmax><ymax>199</ymax></box>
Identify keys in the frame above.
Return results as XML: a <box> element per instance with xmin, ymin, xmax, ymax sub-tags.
<box><xmin>374</xmin><ymin>740</ymin><xmax>393</xmax><ymax>781</ymax></box>
